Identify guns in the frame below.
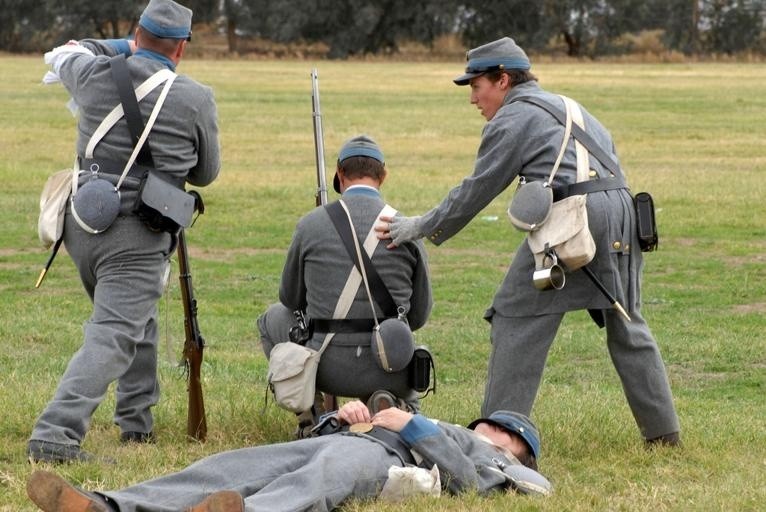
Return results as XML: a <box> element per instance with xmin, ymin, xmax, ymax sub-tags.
<box><xmin>310</xmin><ymin>67</ymin><xmax>327</xmax><ymax>206</ymax></box>
<box><xmin>177</xmin><ymin>230</ymin><xmax>207</xmax><ymax>442</ymax></box>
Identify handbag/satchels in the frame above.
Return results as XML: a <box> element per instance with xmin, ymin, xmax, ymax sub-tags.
<box><xmin>36</xmin><ymin>167</ymin><xmax>76</xmax><ymax>252</ymax></box>
<box><xmin>526</xmin><ymin>193</ymin><xmax>598</xmax><ymax>276</ymax></box>
<box><xmin>264</xmin><ymin>340</ymin><xmax>322</xmax><ymax>415</ymax></box>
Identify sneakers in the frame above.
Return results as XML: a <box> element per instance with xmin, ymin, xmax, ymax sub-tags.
<box><xmin>370</xmin><ymin>388</ymin><xmax>400</xmax><ymax>420</ymax></box>
<box><xmin>644</xmin><ymin>430</ymin><xmax>685</xmax><ymax>454</ymax></box>
<box><xmin>119</xmin><ymin>431</ymin><xmax>155</xmax><ymax>444</ymax></box>
<box><xmin>28</xmin><ymin>438</ymin><xmax>118</xmax><ymax>467</ymax></box>
<box><xmin>25</xmin><ymin>469</ymin><xmax>119</xmax><ymax>512</ymax></box>
<box><xmin>177</xmin><ymin>488</ymin><xmax>245</xmax><ymax>512</ymax></box>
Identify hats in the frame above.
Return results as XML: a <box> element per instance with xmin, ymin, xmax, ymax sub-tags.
<box><xmin>138</xmin><ymin>0</ymin><xmax>194</xmax><ymax>39</ymax></box>
<box><xmin>332</xmin><ymin>135</ymin><xmax>387</xmax><ymax>196</ymax></box>
<box><xmin>465</xmin><ymin>409</ymin><xmax>541</xmax><ymax>466</ymax></box>
<box><xmin>453</xmin><ymin>36</ymin><xmax>533</xmax><ymax>87</ymax></box>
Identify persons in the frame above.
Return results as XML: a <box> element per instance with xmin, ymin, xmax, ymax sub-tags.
<box><xmin>254</xmin><ymin>133</ymin><xmax>434</xmax><ymax>441</ymax></box>
<box><xmin>24</xmin><ymin>0</ymin><xmax>222</xmax><ymax>469</ymax></box>
<box><xmin>374</xmin><ymin>35</ymin><xmax>687</xmax><ymax>455</ymax></box>
<box><xmin>26</xmin><ymin>398</ymin><xmax>541</xmax><ymax>512</ymax></box>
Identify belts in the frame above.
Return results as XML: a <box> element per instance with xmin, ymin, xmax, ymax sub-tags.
<box><xmin>307</xmin><ymin>318</ymin><xmax>387</xmax><ymax>333</ymax></box>
<box><xmin>335</xmin><ymin>421</ymin><xmax>419</xmax><ymax>468</ymax></box>
<box><xmin>79</xmin><ymin>155</ymin><xmax>189</xmax><ymax>192</ymax></box>
<box><xmin>546</xmin><ymin>176</ymin><xmax>629</xmax><ymax>200</ymax></box>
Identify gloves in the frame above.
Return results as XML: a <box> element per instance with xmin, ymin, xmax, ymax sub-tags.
<box><xmin>389</xmin><ymin>214</ymin><xmax>424</xmax><ymax>248</ymax></box>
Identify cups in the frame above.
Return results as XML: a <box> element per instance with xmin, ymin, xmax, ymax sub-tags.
<box><xmin>532</xmin><ymin>254</ymin><xmax>567</xmax><ymax>291</ymax></box>
